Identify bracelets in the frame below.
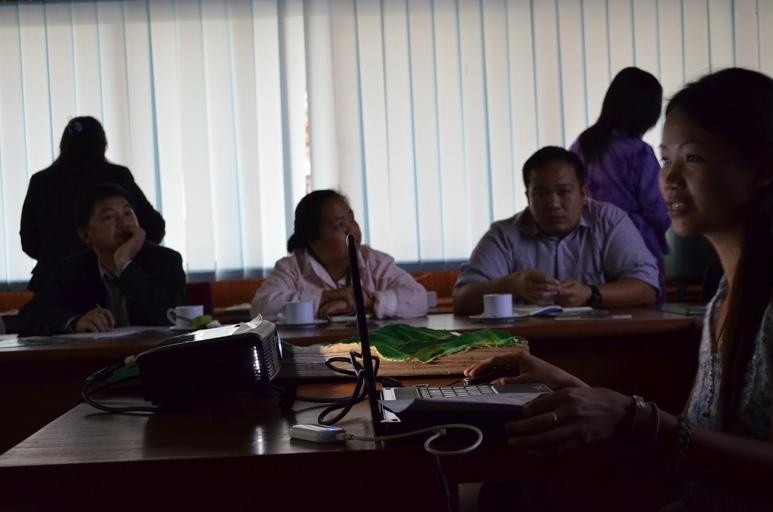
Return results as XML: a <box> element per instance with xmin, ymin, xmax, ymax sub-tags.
<box><xmin>645</xmin><ymin>401</ymin><xmax>660</xmax><ymax>433</ymax></box>
<box><xmin>678</xmin><ymin>415</ymin><xmax>690</xmax><ymax>461</ymax></box>
<box><xmin>633</xmin><ymin>393</ymin><xmax>646</xmax><ymax>433</ymax></box>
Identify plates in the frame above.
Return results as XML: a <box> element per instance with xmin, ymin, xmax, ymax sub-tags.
<box><xmin>167</xmin><ymin>325</ymin><xmax>198</xmax><ymax>330</ymax></box>
<box><xmin>469</xmin><ymin>313</ymin><xmax>529</xmax><ymax>322</ymax></box>
<box><xmin>275</xmin><ymin>317</ymin><xmax>328</xmax><ymax>329</ymax></box>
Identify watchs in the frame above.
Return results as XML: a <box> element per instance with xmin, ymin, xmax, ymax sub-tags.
<box><xmin>588</xmin><ymin>284</ymin><xmax>602</xmax><ymax>309</ymax></box>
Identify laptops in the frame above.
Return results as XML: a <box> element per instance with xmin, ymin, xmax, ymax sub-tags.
<box><xmin>345</xmin><ymin>233</ymin><xmax>556</xmax><ymax>440</ymax></box>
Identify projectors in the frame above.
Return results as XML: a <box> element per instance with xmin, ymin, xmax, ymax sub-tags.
<box><xmin>132</xmin><ymin>313</ymin><xmax>284</xmax><ymax>403</ymax></box>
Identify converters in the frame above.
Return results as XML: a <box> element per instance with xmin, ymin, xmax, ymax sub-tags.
<box><xmin>290</xmin><ymin>423</ymin><xmax>345</xmax><ymax>442</ymax></box>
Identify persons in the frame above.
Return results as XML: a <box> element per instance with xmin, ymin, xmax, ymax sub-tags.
<box><xmin>452</xmin><ymin>146</ymin><xmax>662</xmax><ymax>315</ymax></box>
<box><xmin>21</xmin><ymin>117</ymin><xmax>165</xmax><ymax>296</ymax></box>
<box><xmin>15</xmin><ymin>184</ymin><xmax>186</xmax><ymax>336</ymax></box>
<box><xmin>462</xmin><ymin>68</ymin><xmax>773</xmax><ymax>501</ymax></box>
<box><xmin>250</xmin><ymin>190</ymin><xmax>428</xmax><ymax>322</ymax></box>
<box><xmin>569</xmin><ymin>67</ymin><xmax>672</xmax><ymax>305</ymax></box>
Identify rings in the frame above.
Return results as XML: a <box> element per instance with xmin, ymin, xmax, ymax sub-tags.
<box><xmin>551</xmin><ymin>410</ymin><xmax>560</xmax><ymax>429</ymax></box>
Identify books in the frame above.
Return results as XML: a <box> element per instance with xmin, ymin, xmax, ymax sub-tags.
<box><xmin>513</xmin><ymin>304</ymin><xmax>593</xmax><ymax>317</ymax></box>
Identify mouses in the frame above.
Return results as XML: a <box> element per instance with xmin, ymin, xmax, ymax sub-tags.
<box><xmin>463</xmin><ymin>364</ymin><xmax>520</xmax><ymax>386</ymax></box>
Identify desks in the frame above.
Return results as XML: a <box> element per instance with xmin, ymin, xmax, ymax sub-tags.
<box><xmin>0</xmin><ymin>377</ymin><xmax>554</xmax><ymax>512</ymax></box>
<box><xmin>0</xmin><ymin>302</ymin><xmax>705</xmax><ymax>451</ymax></box>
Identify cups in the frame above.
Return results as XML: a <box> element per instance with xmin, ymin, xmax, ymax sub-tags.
<box><xmin>280</xmin><ymin>300</ymin><xmax>314</xmax><ymax>324</ymax></box>
<box><xmin>167</xmin><ymin>305</ymin><xmax>203</xmax><ymax>327</ymax></box>
<box><xmin>483</xmin><ymin>293</ymin><xmax>513</xmax><ymax>317</ymax></box>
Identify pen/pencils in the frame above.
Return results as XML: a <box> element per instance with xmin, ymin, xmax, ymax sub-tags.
<box><xmin>326</xmin><ymin>315</ymin><xmax>330</xmax><ymax>322</ymax></box>
<box><xmin>96</xmin><ymin>304</ymin><xmax>103</xmax><ymax>314</ymax></box>
